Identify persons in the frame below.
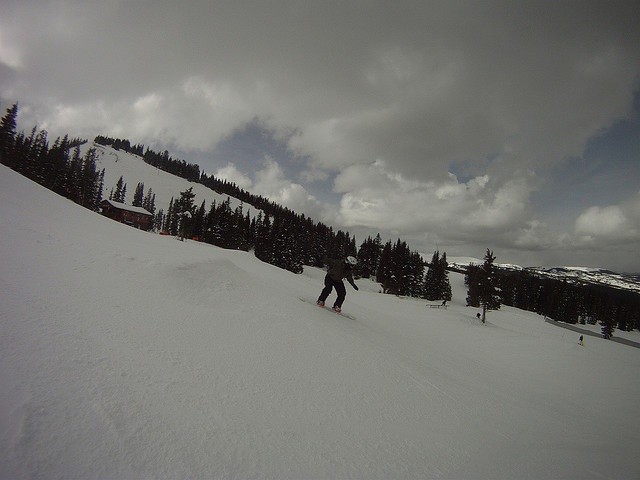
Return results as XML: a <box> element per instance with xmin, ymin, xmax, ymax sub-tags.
<box><xmin>316</xmin><ymin>255</ymin><xmax>359</xmax><ymax>313</ymax></box>
<box><xmin>578</xmin><ymin>334</ymin><xmax>583</xmax><ymax>345</ymax></box>
<box><xmin>441</xmin><ymin>300</ymin><xmax>447</xmax><ymax>306</ymax></box>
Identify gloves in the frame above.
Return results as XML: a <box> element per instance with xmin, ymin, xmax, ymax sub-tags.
<box><xmin>352</xmin><ymin>284</ymin><xmax>359</xmax><ymax>291</ymax></box>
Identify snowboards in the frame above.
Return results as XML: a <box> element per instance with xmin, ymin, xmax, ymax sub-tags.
<box><xmin>298</xmin><ymin>294</ymin><xmax>356</xmax><ymax>320</ymax></box>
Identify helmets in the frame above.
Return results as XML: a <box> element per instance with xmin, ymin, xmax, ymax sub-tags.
<box><xmin>345</xmin><ymin>256</ymin><xmax>358</xmax><ymax>266</ymax></box>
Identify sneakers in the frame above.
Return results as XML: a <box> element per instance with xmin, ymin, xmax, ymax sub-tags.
<box><xmin>332</xmin><ymin>305</ymin><xmax>341</xmax><ymax>312</ymax></box>
<box><xmin>316</xmin><ymin>300</ymin><xmax>325</xmax><ymax>306</ymax></box>
